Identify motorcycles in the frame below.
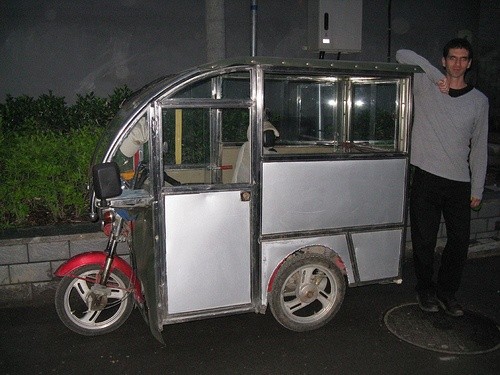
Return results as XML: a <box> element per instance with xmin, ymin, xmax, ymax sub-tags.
<box><xmin>53</xmin><ymin>56</ymin><xmax>414</xmax><ymax>347</ymax></box>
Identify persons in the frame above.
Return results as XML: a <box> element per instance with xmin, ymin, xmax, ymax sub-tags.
<box><xmin>396</xmin><ymin>39</ymin><xmax>489</xmax><ymax>317</ymax></box>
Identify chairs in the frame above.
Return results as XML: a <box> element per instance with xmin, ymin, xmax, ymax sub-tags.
<box><xmin>231</xmin><ymin>121</ymin><xmax>279</xmax><ymax>184</ymax></box>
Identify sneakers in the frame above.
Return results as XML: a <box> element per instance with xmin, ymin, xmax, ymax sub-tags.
<box><xmin>417</xmin><ymin>292</ymin><xmax>439</xmax><ymax>312</ymax></box>
<box><xmin>435</xmin><ymin>293</ymin><xmax>464</xmax><ymax>316</ymax></box>
